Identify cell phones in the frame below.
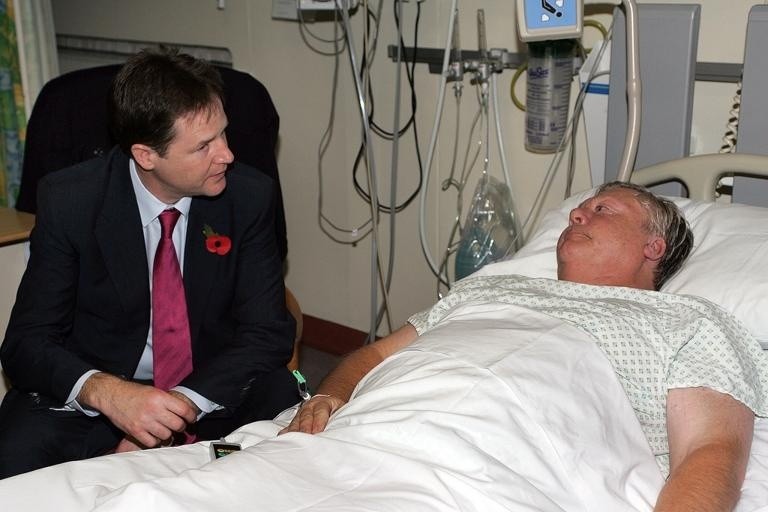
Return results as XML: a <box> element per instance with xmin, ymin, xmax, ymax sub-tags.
<box><xmin>208</xmin><ymin>441</ymin><xmax>241</xmax><ymax>459</ymax></box>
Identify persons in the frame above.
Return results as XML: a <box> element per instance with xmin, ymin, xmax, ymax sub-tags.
<box><xmin>276</xmin><ymin>176</ymin><xmax>768</xmax><ymax>511</ymax></box>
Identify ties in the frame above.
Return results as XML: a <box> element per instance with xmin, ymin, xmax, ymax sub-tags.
<box><xmin>150</xmin><ymin>208</ymin><xmax>193</xmax><ymax>393</ymax></box>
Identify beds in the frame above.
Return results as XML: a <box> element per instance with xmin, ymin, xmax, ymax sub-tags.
<box><xmin>1</xmin><ymin>149</ymin><xmax>766</xmax><ymax>510</ymax></box>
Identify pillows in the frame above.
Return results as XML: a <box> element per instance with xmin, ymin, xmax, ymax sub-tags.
<box><xmin>465</xmin><ymin>184</ymin><xmax>768</xmax><ymax>349</ymax></box>
